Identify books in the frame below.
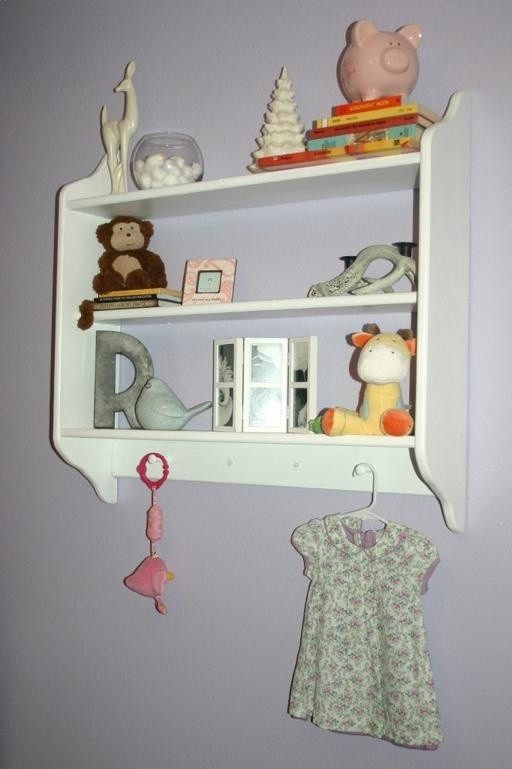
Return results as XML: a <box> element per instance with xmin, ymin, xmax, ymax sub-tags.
<box><xmin>254</xmin><ymin>92</ymin><xmax>441</xmax><ymax>164</ymax></box>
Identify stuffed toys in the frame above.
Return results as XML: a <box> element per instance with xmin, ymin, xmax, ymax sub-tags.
<box><xmin>123</xmin><ymin>504</ymin><xmax>176</xmax><ymax>616</ymax></box>
<box><xmin>310</xmin><ymin>322</ymin><xmax>419</xmax><ymax>437</ymax></box>
<box><xmin>91</xmin><ymin>213</ymin><xmax>169</xmax><ymax>293</ymax></box>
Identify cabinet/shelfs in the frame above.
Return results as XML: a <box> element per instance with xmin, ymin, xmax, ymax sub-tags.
<box><xmin>49</xmin><ymin>91</ymin><xmax>469</xmax><ymax>533</ymax></box>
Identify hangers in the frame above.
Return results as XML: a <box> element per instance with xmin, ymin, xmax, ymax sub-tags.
<box><xmin>339</xmin><ymin>462</ymin><xmax>390</xmax><ymax>525</ymax></box>
